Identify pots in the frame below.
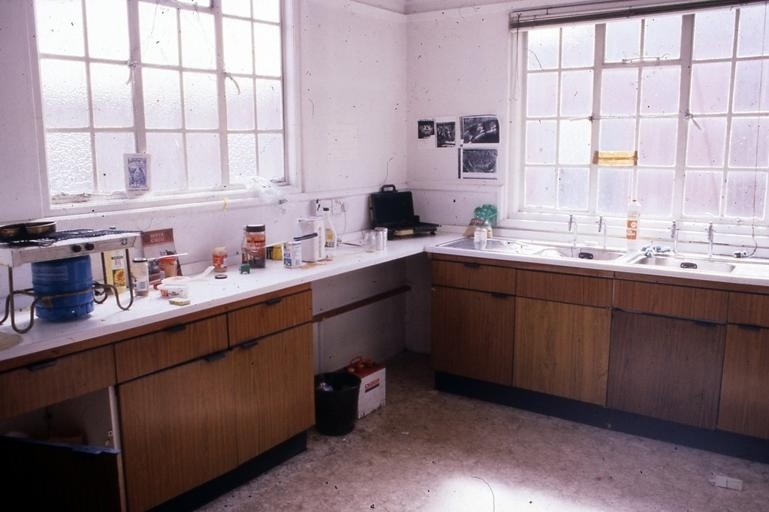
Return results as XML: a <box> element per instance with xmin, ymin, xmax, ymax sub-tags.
<box><xmin>1</xmin><ymin>219</ymin><xmax>57</xmax><ymax>243</ymax></box>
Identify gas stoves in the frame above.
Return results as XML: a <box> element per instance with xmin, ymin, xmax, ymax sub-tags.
<box><xmin>0</xmin><ymin>228</ymin><xmax>142</xmax><ymax>269</ymax></box>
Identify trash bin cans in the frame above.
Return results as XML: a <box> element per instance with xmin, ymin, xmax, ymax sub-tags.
<box><xmin>313</xmin><ymin>372</ymin><xmax>361</xmax><ymax>436</ymax></box>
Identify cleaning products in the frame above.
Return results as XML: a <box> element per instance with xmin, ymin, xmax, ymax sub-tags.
<box><xmin>625</xmin><ymin>196</ymin><xmax>641</xmax><ymax>240</ymax></box>
<box><xmin>320</xmin><ymin>207</ymin><xmax>338</xmax><ymax>257</ymax></box>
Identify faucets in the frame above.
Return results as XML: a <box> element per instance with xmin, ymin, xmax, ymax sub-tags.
<box><xmin>568</xmin><ymin>215</ymin><xmax>579</xmax><ymax>245</ymax></box>
<box><xmin>705</xmin><ymin>223</ymin><xmax>717</xmax><ymax>257</ymax></box>
<box><xmin>667</xmin><ymin>223</ymin><xmax>679</xmax><ymax>250</ymax></box>
<box><xmin>594</xmin><ymin>217</ymin><xmax>607</xmax><ymax>246</ymax></box>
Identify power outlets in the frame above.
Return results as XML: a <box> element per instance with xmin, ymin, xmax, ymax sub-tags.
<box><xmin>332</xmin><ymin>198</ymin><xmax>348</xmax><ymax>216</ymax></box>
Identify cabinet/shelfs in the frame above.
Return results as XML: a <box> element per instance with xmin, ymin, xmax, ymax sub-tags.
<box><xmin>606</xmin><ymin>279</ymin><xmax>769</xmax><ymax>441</ymax></box>
<box><xmin>429</xmin><ymin>262</ymin><xmax>613</xmax><ymax>408</ymax></box>
<box><xmin>113</xmin><ymin>288</ymin><xmax>316</xmax><ymax>512</ymax></box>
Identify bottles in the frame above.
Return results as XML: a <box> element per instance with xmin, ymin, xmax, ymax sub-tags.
<box><xmin>323</xmin><ymin>207</ymin><xmax>338</xmax><ymax>248</ymax></box>
<box><xmin>374</xmin><ymin>226</ymin><xmax>389</xmax><ymax>252</ymax></box>
<box><xmin>148</xmin><ymin>249</ymin><xmax>179</xmax><ymax>286</ymax></box>
<box><xmin>110</xmin><ymin>226</ymin><xmax>127</xmax><ymax>288</ymax></box>
<box><xmin>363</xmin><ymin>230</ymin><xmax>376</xmax><ymax>254</ymax></box>
<box><xmin>474</xmin><ymin>228</ymin><xmax>487</xmax><ymax>250</ymax></box>
<box><xmin>626</xmin><ymin>200</ymin><xmax>642</xmax><ymax>248</ymax></box>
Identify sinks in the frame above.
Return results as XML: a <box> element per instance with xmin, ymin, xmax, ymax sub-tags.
<box><xmin>534</xmin><ymin>246</ymin><xmax>627</xmax><ymax>262</ymax></box>
<box><xmin>634</xmin><ymin>257</ymin><xmax>735</xmax><ymax>274</ymax></box>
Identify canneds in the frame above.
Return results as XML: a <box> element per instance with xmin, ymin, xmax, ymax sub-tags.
<box><xmin>246</xmin><ymin>224</ymin><xmax>266</xmax><ymax>267</ymax></box>
<box><xmin>283</xmin><ymin>241</ymin><xmax>302</xmax><ymax>268</ymax></box>
<box><xmin>159</xmin><ymin>257</ymin><xmax>177</xmax><ymax>278</ymax></box>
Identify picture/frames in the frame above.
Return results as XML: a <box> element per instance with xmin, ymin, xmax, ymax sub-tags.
<box><xmin>123</xmin><ymin>153</ymin><xmax>153</xmax><ymax>194</ymax></box>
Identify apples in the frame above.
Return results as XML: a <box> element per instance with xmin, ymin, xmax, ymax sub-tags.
<box><xmin>347</xmin><ymin>355</ymin><xmax>374</xmax><ymax>374</ymax></box>
